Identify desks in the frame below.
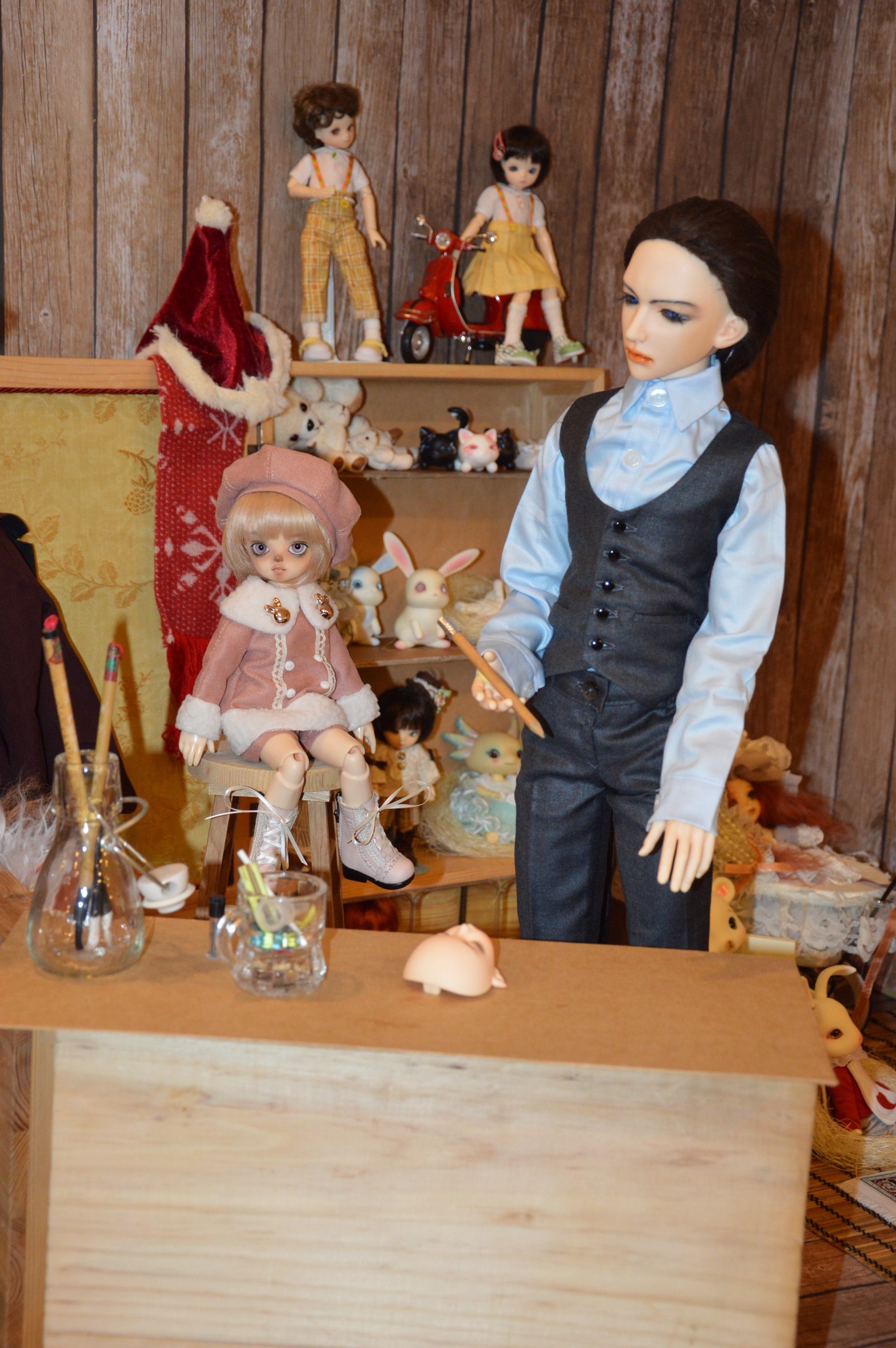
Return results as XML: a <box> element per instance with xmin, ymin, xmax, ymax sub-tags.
<box><xmin>1</xmin><ymin>904</ymin><xmax>842</xmax><ymax>1347</ymax></box>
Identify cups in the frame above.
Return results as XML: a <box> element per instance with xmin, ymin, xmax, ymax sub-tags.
<box><xmin>214</xmin><ymin>871</ymin><xmax>326</xmax><ymax>998</ymax></box>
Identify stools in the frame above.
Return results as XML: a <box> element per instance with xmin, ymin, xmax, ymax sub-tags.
<box><xmin>187</xmin><ymin>738</ymin><xmax>344</xmax><ymax>929</ymax></box>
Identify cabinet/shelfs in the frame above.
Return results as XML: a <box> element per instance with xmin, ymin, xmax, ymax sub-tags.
<box><xmin>284</xmin><ymin>349</ymin><xmax>608</xmax><ymax>946</ymax></box>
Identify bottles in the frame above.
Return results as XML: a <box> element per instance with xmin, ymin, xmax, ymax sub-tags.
<box><xmin>27</xmin><ymin>748</ymin><xmax>145</xmax><ymax>976</ymax></box>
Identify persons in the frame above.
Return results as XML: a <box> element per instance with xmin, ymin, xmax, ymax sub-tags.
<box><xmin>727</xmin><ymin>764</ymin><xmax>848</xmax><ymax>849</ymax></box>
<box><xmin>367</xmin><ymin>667</ymin><xmax>451</xmax><ymax>869</ymax></box>
<box><xmin>286</xmin><ymin>83</ymin><xmax>389</xmax><ymax>364</ymax></box>
<box><xmin>471</xmin><ymin>198</ymin><xmax>788</xmax><ymax>953</ymax></box>
<box><xmin>462</xmin><ymin>125</ymin><xmax>586</xmax><ymax>367</ymax></box>
<box><xmin>173</xmin><ymin>443</ymin><xmax>418</xmax><ymax>890</ymax></box>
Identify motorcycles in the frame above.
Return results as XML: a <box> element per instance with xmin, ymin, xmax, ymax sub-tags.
<box><xmin>395</xmin><ymin>215</ymin><xmax>553</xmax><ymax>368</ymax></box>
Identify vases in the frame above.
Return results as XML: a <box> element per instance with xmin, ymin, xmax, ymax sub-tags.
<box><xmin>24</xmin><ymin>745</ymin><xmax>170</xmax><ymax>981</ymax></box>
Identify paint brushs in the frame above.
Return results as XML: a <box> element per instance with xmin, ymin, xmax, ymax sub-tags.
<box><xmin>40</xmin><ymin>614</ymin><xmax>116</xmax><ymax>953</ymax></box>
<box><xmin>71</xmin><ymin>638</ymin><xmax>127</xmax><ymax>951</ymax></box>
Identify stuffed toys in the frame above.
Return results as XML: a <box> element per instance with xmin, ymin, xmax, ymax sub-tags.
<box><xmin>273</xmin><ymin>375</ymin><xmax>419</xmax><ymax>475</ymax></box>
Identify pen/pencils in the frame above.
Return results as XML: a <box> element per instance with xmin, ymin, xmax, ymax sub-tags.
<box><xmin>436</xmin><ymin>614</ymin><xmax>546</xmax><ymax>739</ymax></box>
